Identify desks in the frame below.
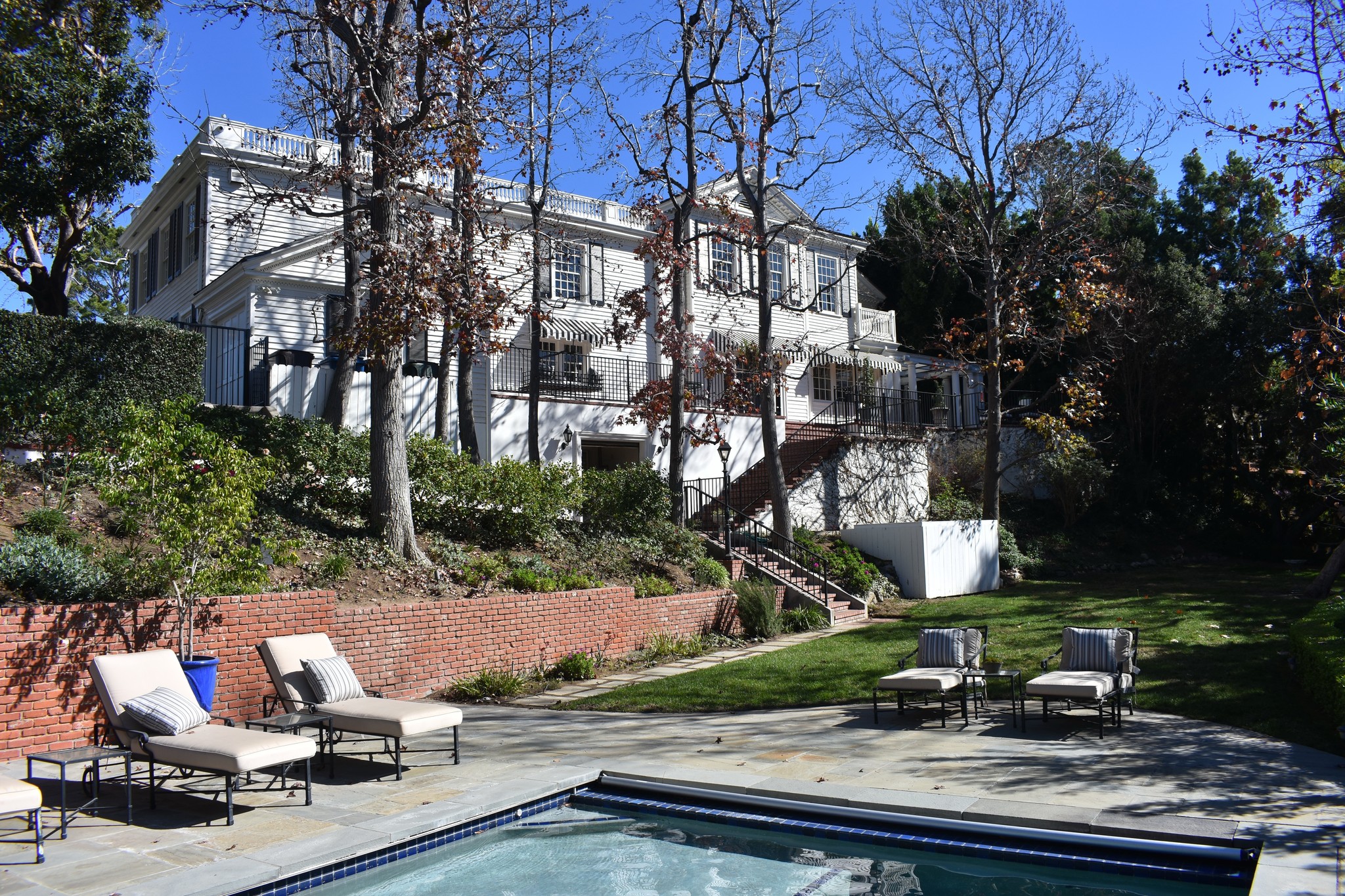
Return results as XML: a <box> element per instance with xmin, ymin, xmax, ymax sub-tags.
<box><xmin>962</xmin><ymin>670</ymin><xmax>1025</xmax><ymax>728</ymax></box>
<box><xmin>26</xmin><ymin>745</ymin><xmax>132</xmax><ymax>839</ymax></box>
<box><xmin>245</xmin><ymin>712</ymin><xmax>335</xmax><ymax>785</ymax></box>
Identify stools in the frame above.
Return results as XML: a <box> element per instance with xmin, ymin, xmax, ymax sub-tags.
<box><xmin>960</xmin><ymin>692</ymin><xmax>984</xmax><ymax>709</ymax></box>
<box><xmin>0</xmin><ymin>775</ymin><xmax>45</xmax><ymax>863</ymax></box>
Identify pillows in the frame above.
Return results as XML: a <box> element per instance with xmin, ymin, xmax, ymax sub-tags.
<box><xmin>298</xmin><ymin>655</ymin><xmax>367</xmax><ymax>704</ymax></box>
<box><xmin>1068</xmin><ymin>627</ymin><xmax>1118</xmax><ymax>673</ymax></box>
<box><xmin>118</xmin><ymin>687</ymin><xmax>212</xmax><ymax>735</ymax></box>
<box><xmin>920</xmin><ymin>627</ymin><xmax>968</xmax><ymax>669</ymax></box>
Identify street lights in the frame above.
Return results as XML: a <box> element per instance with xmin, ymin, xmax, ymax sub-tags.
<box><xmin>716</xmin><ymin>433</ymin><xmax>735</xmax><ymax>563</ymax></box>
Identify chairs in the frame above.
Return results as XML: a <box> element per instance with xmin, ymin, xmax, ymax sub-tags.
<box><xmin>255</xmin><ymin>631</ymin><xmax>463</xmax><ymax>781</ymax></box>
<box><xmin>1026</xmin><ymin>626</ymin><xmax>1140</xmax><ymax>739</ymax></box>
<box><xmin>873</xmin><ymin>625</ymin><xmax>989</xmax><ymax>728</ymax></box>
<box><xmin>83</xmin><ymin>649</ymin><xmax>318</xmax><ymax>826</ymax></box>
